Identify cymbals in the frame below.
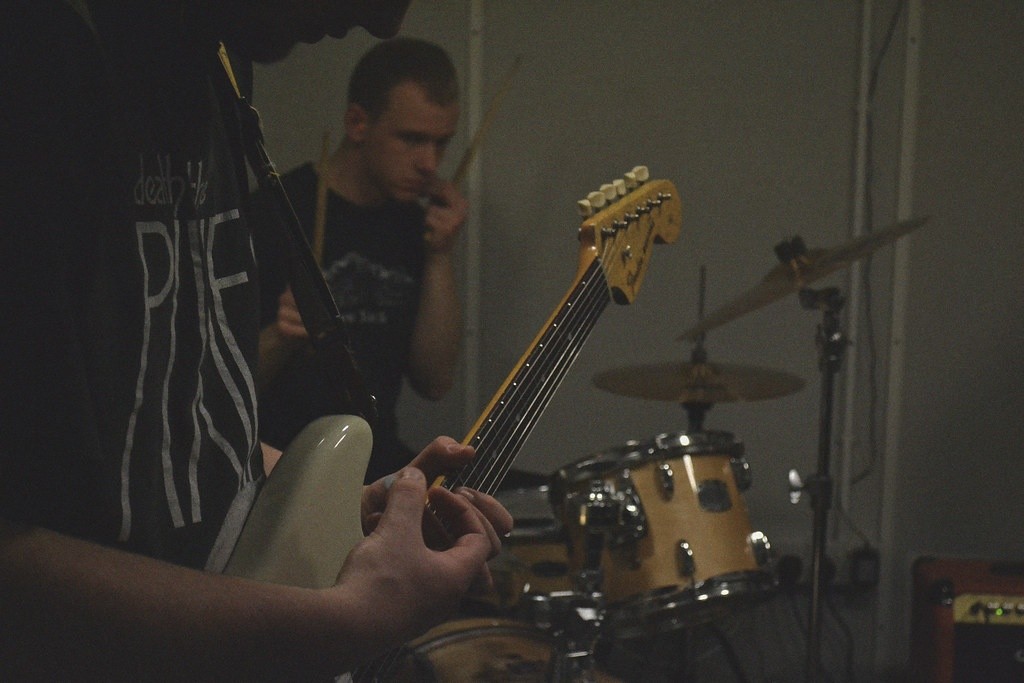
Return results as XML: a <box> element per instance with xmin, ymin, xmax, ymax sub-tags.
<box><xmin>589</xmin><ymin>212</ymin><xmax>933</xmax><ymax>406</ymax></box>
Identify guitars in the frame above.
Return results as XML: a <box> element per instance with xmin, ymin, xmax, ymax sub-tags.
<box><xmin>208</xmin><ymin>164</ymin><xmax>683</xmax><ymax>590</ymax></box>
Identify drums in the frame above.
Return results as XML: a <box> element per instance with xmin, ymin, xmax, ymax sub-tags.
<box><xmin>352</xmin><ymin>434</ymin><xmax>776</xmax><ymax>683</ymax></box>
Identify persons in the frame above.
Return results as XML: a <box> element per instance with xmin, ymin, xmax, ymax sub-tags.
<box><xmin>0</xmin><ymin>0</ymin><xmax>514</xmax><ymax>683</ymax></box>
<box><xmin>250</xmin><ymin>37</ymin><xmax>469</xmax><ymax>498</ymax></box>
